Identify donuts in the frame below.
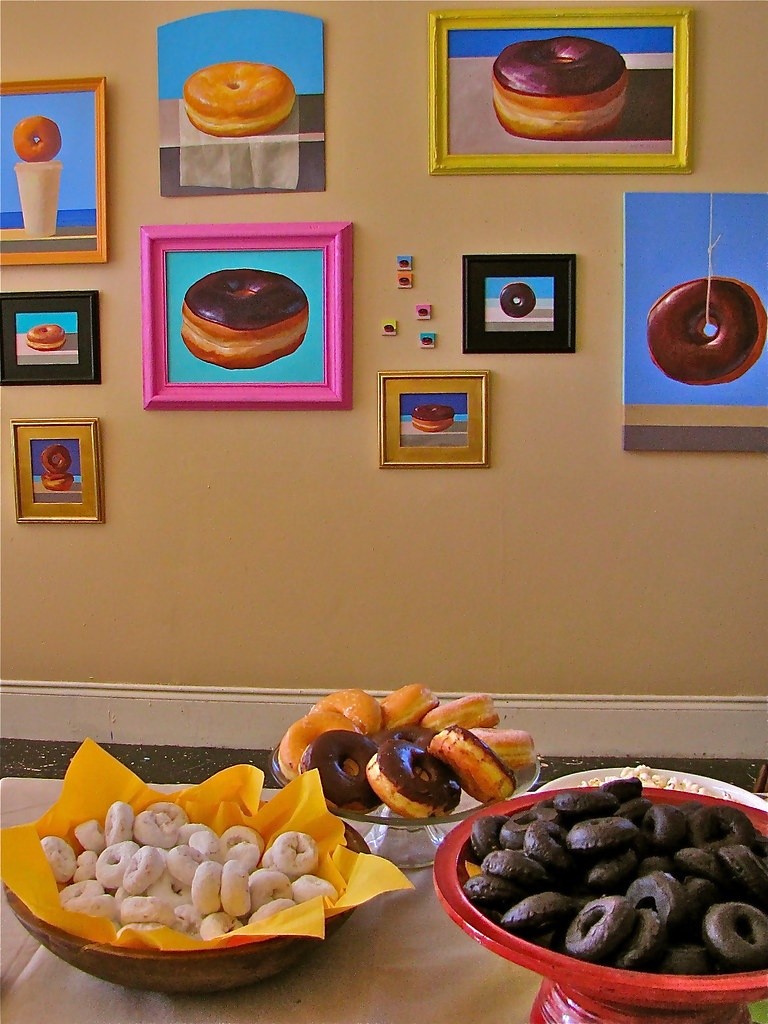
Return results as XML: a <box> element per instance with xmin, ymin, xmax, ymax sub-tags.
<box><xmin>39</xmin><ymin>800</ymin><xmax>339</xmax><ymax>939</ymax></box>
<box><xmin>277</xmin><ymin>683</ymin><xmax>534</xmax><ymax>819</ymax></box>
<box><xmin>463</xmin><ymin>778</ymin><xmax>768</xmax><ymax>975</ymax></box>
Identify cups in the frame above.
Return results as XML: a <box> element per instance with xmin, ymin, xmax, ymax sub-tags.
<box><xmin>14</xmin><ymin>160</ymin><xmax>62</xmax><ymax>236</ymax></box>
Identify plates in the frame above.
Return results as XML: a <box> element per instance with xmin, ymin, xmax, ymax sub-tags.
<box><xmin>533</xmin><ymin>765</ymin><xmax>768</xmax><ymax>811</ymax></box>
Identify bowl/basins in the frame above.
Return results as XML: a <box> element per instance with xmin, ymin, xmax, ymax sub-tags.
<box><xmin>4</xmin><ymin>820</ymin><xmax>372</xmax><ymax>992</ymax></box>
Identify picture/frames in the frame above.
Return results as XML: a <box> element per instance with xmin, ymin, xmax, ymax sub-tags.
<box><xmin>1</xmin><ymin>289</ymin><xmax>101</xmax><ymax>385</ymax></box>
<box><xmin>9</xmin><ymin>415</ymin><xmax>105</xmax><ymax>526</ymax></box>
<box><xmin>463</xmin><ymin>254</ymin><xmax>575</xmax><ymax>356</ymax></box>
<box><xmin>428</xmin><ymin>8</ymin><xmax>693</xmax><ymax>178</ymax></box>
<box><xmin>1</xmin><ymin>76</ymin><xmax>109</xmax><ymax>263</ymax></box>
<box><xmin>139</xmin><ymin>222</ymin><xmax>355</xmax><ymax>413</ymax></box>
<box><xmin>377</xmin><ymin>369</ymin><xmax>490</xmax><ymax>469</ymax></box>
